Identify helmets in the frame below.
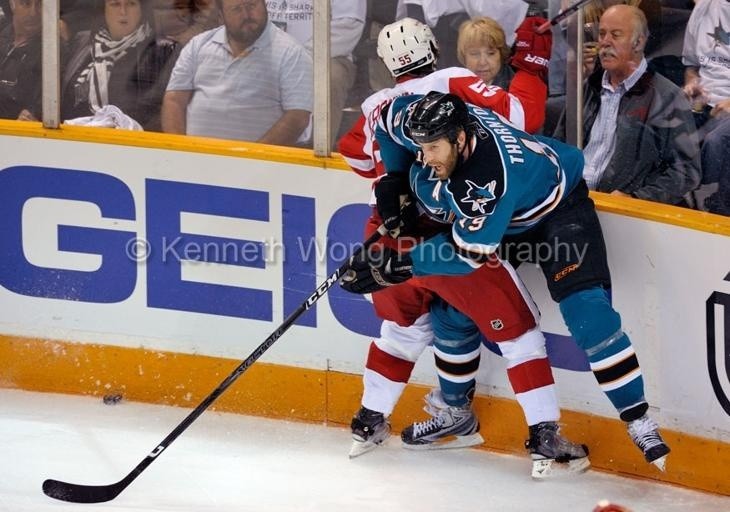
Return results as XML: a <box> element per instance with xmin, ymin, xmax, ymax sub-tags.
<box><xmin>376</xmin><ymin>18</ymin><xmax>440</xmax><ymax>83</ymax></box>
<box><xmin>405</xmin><ymin>91</ymin><xmax>478</xmax><ymax>144</ymax></box>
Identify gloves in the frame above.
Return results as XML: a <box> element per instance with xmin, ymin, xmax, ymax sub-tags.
<box><xmin>510</xmin><ymin>17</ymin><xmax>552</xmax><ymax>76</ymax></box>
<box><xmin>339</xmin><ymin>255</ymin><xmax>412</xmax><ymax>294</ymax></box>
<box><xmin>375</xmin><ymin>171</ymin><xmax>420</xmax><ymax>239</ymax></box>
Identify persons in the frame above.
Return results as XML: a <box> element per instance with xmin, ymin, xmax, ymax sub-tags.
<box><xmin>2</xmin><ymin>2</ymin><xmax>730</xmax><ymax>216</ymax></box>
<box><xmin>337</xmin><ymin>16</ymin><xmax>590</xmax><ymax>460</ymax></box>
<box><xmin>338</xmin><ymin>91</ymin><xmax>670</xmax><ymax>464</ymax></box>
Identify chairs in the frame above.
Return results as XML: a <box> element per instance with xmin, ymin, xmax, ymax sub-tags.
<box><xmin>648</xmin><ymin>54</ymin><xmax>699</xmax><ymax>210</ymax></box>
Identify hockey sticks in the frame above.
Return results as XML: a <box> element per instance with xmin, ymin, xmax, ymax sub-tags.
<box><xmin>42</xmin><ymin>192</ymin><xmax>417</xmax><ymax>504</ymax></box>
<box><xmin>342</xmin><ymin>0</ymin><xmax>588</xmax><ymax>112</ymax></box>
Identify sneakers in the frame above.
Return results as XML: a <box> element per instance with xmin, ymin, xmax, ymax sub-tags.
<box><xmin>401</xmin><ymin>388</ymin><xmax>480</xmax><ymax>446</ymax></box>
<box><xmin>525</xmin><ymin>421</ymin><xmax>589</xmax><ymax>463</ymax></box>
<box><xmin>626</xmin><ymin>413</ymin><xmax>671</xmax><ymax>463</ymax></box>
<box><xmin>351</xmin><ymin>405</ymin><xmax>392</xmax><ymax>444</ymax></box>
